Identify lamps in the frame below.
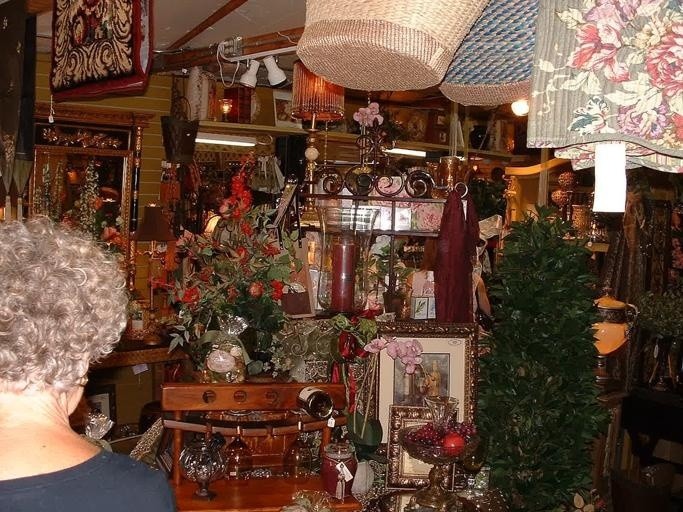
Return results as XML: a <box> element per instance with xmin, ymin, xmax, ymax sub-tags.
<box><xmin>215</xmin><ymin>36</ymin><xmax>299</xmax><ymax>89</ymax></box>
<box><xmin>131</xmin><ymin>205</ymin><xmax>178</xmax><ymax>287</ymax></box>
<box><xmin>292</xmin><ymin>58</ymin><xmax>345</xmax><ymax>122</ymax></box>
<box><xmin>594</xmin><ymin>277</ymin><xmax>639</xmax><ymax>392</ymax></box>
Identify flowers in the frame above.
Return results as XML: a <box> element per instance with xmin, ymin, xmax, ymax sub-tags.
<box><xmin>146</xmin><ymin>152</ymin><xmax>303</xmax><ymax>352</ymax></box>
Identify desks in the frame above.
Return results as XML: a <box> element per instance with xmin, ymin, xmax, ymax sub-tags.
<box><xmin>588</xmin><ymin>392</ymin><xmax>629</xmax><ymax>503</ymax></box>
<box><xmin>89</xmin><ymin>347</ymin><xmax>190</xmax><ymax>401</ymax></box>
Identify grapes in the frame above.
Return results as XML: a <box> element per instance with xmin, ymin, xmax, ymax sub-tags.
<box><xmin>404</xmin><ymin>422</ymin><xmax>478</xmax><ymax>456</ymax></box>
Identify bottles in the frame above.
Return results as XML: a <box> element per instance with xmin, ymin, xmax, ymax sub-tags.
<box><xmin>296</xmin><ymin>387</ymin><xmax>333</xmax><ymax>418</ymax></box>
<box><xmin>400</xmin><ymin>359</ymin><xmax>440</xmax><ymax>402</ymax></box>
<box><xmin>319</xmin><ymin>442</ymin><xmax>357</xmax><ymax>497</ymax></box>
<box><xmin>158</xmin><ymin>160</ymin><xmax>180</xmax><ymax>184</ymax></box>
<box><xmin>571</xmin><ymin>203</ymin><xmax>587</xmax><ymax>230</ymax></box>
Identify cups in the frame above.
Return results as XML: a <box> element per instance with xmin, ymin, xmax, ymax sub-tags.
<box><xmin>313</xmin><ymin>206</ymin><xmax>380</xmax><ymax>310</ymax></box>
<box><xmin>590</xmin><ymin>235</ymin><xmax>597</xmax><ymax>243</ymax></box>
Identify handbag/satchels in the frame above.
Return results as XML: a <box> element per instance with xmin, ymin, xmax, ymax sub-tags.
<box><xmin>159</xmin><ymin>98</ymin><xmax>200</xmax><ymax>164</ymax></box>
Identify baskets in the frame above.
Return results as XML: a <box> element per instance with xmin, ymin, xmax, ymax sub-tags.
<box><xmin>437</xmin><ymin>0</ymin><xmax>545</xmax><ymax>109</ymax></box>
<box><xmin>294</xmin><ymin>0</ymin><xmax>492</xmax><ymax>93</ymax></box>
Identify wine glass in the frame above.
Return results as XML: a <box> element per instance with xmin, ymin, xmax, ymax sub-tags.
<box><xmin>282</xmin><ymin>408</ymin><xmax>313</xmax><ymax>484</ymax></box>
<box><xmin>177</xmin><ymin>439</ymin><xmax>229</xmax><ymax>502</ymax></box>
<box><xmin>396</xmin><ymin>425</ymin><xmax>478</xmax><ymax>511</ymax></box>
<box><xmin>218</xmin><ymin>98</ymin><xmax>232</xmax><ymax>122</ymax></box>
<box><xmin>223</xmin><ymin>409</ymin><xmax>251</xmax><ymax>486</ymax></box>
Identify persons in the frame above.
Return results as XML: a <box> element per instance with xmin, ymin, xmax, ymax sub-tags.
<box><xmin>0</xmin><ymin>211</ymin><xmax>179</xmax><ymax>510</ymax></box>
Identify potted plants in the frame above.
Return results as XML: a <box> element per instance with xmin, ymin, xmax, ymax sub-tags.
<box><xmin>356</xmin><ymin>239</ymin><xmax>417</xmax><ymax>319</ymax></box>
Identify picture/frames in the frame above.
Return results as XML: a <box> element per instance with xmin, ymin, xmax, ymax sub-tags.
<box><xmin>31</xmin><ymin>145</ymin><xmax>134</xmax><ymax>273</ymax></box>
<box><xmin>386</xmin><ymin>405</ymin><xmax>459</xmax><ymax>493</ymax></box>
<box><xmin>372</xmin><ymin>322</ymin><xmax>480</xmax><ymax>461</ymax></box>
<box><xmin>85</xmin><ymin>384</ymin><xmax>117</xmax><ymax>429</ymax></box>
